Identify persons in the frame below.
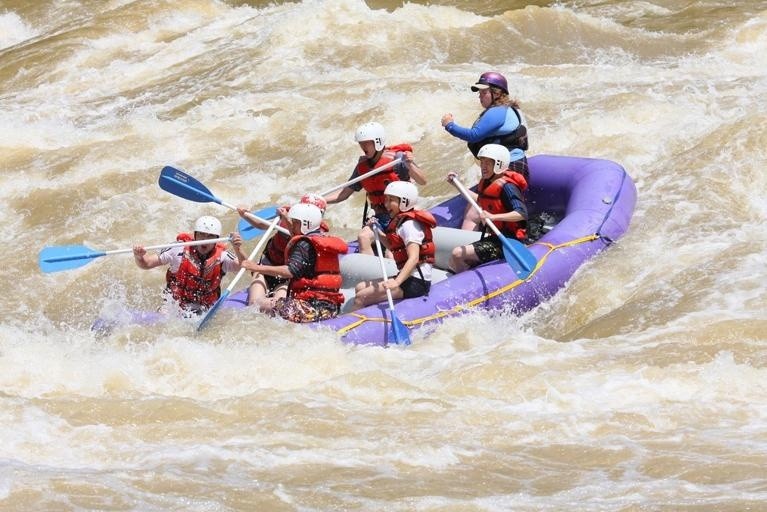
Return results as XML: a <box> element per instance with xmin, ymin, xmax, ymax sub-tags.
<box><xmin>440</xmin><ymin>70</ymin><xmax>528</xmax><ymax>233</ymax></box>
<box><xmin>240</xmin><ymin>203</ymin><xmax>348</xmax><ymax>324</ymax></box>
<box><xmin>350</xmin><ymin>180</ymin><xmax>436</xmax><ymax>314</ymax></box>
<box><xmin>133</xmin><ymin>214</ymin><xmax>249</xmax><ymax>318</ymax></box>
<box><xmin>236</xmin><ymin>191</ymin><xmax>328</xmax><ymax>309</ymax></box>
<box><xmin>321</xmin><ymin>121</ymin><xmax>428</xmax><ymax>256</ymax></box>
<box><xmin>445</xmin><ymin>144</ymin><xmax>528</xmax><ymax>274</ymax></box>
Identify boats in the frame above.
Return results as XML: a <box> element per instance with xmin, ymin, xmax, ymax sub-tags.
<box><xmin>87</xmin><ymin>152</ymin><xmax>637</xmax><ymax>351</ymax></box>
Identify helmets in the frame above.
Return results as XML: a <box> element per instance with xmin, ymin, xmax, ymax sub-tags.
<box><xmin>300</xmin><ymin>192</ymin><xmax>326</xmax><ymax>218</ymax></box>
<box><xmin>193</xmin><ymin>216</ymin><xmax>221</xmax><ymax>239</ymax></box>
<box><xmin>382</xmin><ymin>180</ymin><xmax>418</xmax><ymax>213</ymax></box>
<box><xmin>470</xmin><ymin>71</ymin><xmax>509</xmax><ymax>98</ymax></box>
<box><xmin>285</xmin><ymin>204</ymin><xmax>321</xmax><ymax>236</ymax></box>
<box><xmin>354</xmin><ymin>122</ymin><xmax>388</xmax><ymax>152</ymax></box>
<box><xmin>476</xmin><ymin>142</ymin><xmax>509</xmax><ymax>175</ymax></box>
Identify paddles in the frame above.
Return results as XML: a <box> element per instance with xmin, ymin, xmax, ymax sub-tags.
<box><xmin>451</xmin><ymin>176</ymin><xmax>537</xmax><ymax>280</ymax></box>
<box><xmin>196</xmin><ymin>217</ymin><xmax>280</xmax><ymax>331</ymax></box>
<box><xmin>39</xmin><ymin>237</ymin><xmax>233</xmax><ymax>272</ymax></box>
<box><xmin>238</xmin><ymin>151</ymin><xmax>406</xmax><ymax>241</ymax></box>
<box><xmin>370</xmin><ymin>217</ymin><xmax>410</xmax><ymax>345</ymax></box>
<box><xmin>159</xmin><ymin>166</ymin><xmax>290</xmax><ymax>236</ymax></box>
